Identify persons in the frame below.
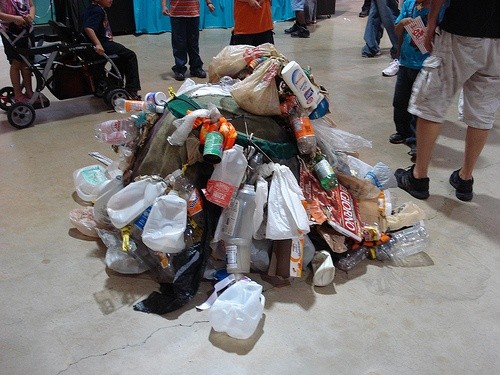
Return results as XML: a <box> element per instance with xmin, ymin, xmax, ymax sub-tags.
<box><xmin>0</xmin><ymin>0</ymin><xmax>35</xmax><ymax>103</ymax></box>
<box><xmin>285</xmin><ymin>0</ymin><xmax>317</xmax><ymax>38</ymax></box>
<box><xmin>81</xmin><ymin>0</ymin><xmax>142</xmax><ymax>100</ymax></box>
<box><xmin>161</xmin><ymin>0</ymin><xmax>215</xmax><ymax>81</ymax></box>
<box><xmin>359</xmin><ymin>0</ymin><xmax>500</xmax><ymax>201</ymax></box>
<box><xmin>230</xmin><ymin>0</ymin><xmax>275</xmax><ymax>47</ymax></box>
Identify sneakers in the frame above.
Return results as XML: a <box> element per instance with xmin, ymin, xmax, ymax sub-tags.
<box><xmin>285</xmin><ymin>23</ymin><xmax>307</xmax><ymax>32</ymax></box>
<box><xmin>394</xmin><ymin>164</ymin><xmax>429</xmax><ymax>199</ymax></box>
<box><xmin>449</xmin><ymin>168</ymin><xmax>474</xmax><ymax>201</ymax></box>
<box><xmin>190</xmin><ymin>68</ymin><xmax>206</xmax><ymax>78</ymax></box>
<box><xmin>382</xmin><ymin>59</ymin><xmax>399</xmax><ymax>76</ymax></box>
<box><xmin>291</xmin><ymin>26</ymin><xmax>310</xmax><ymax>37</ymax></box>
<box><xmin>175</xmin><ymin>70</ymin><xmax>185</xmax><ymax>80</ymax></box>
<box><xmin>359</xmin><ymin>9</ymin><xmax>369</xmax><ymax>17</ymax></box>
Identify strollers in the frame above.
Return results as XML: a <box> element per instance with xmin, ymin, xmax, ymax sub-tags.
<box><xmin>0</xmin><ymin>16</ymin><xmax>143</xmax><ymax>128</ymax></box>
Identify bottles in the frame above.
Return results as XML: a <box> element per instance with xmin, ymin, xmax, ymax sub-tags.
<box><xmin>364</xmin><ymin>162</ymin><xmax>392</xmax><ymax>190</ymax></box>
<box><xmin>290</xmin><ymin>105</ymin><xmax>317</xmax><ymax>154</ymax></box>
<box><xmin>311</xmin><ymin>250</ymin><xmax>335</xmax><ymax>287</ymax></box>
<box><xmin>205</xmin><ymin>144</ymin><xmax>248</xmax><ymax>209</ymax></box>
<box><xmin>333</xmin><ymin>246</ymin><xmax>369</xmax><ymax>270</ymax></box>
<box><xmin>129</xmin><ymin>197</ymin><xmax>158</xmax><ymax>240</ymax></box>
<box><xmin>94</xmin><ymin>117</ymin><xmax>138</xmax><ymax>146</ymax></box>
<box><xmin>202</xmin><ymin>131</ymin><xmax>224</xmax><ymax>163</ymax></box>
<box><xmin>106</xmin><ymin>175</ymin><xmax>176</xmax><ymax>230</ymax></box>
<box><xmin>226</xmin><ymin>184</ymin><xmax>257</xmax><ymax>238</ymax></box>
<box><xmin>311</xmin><ymin>153</ymin><xmax>339</xmax><ymax>190</ymax></box>
<box><xmin>114</xmin><ymin>98</ymin><xmax>164</xmax><ymax>115</ymax></box>
<box><xmin>376</xmin><ymin>225</ymin><xmax>429</xmax><ymax>261</ymax></box>
<box><xmin>172</xmin><ymin>168</ymin><xmax>206</xmax><ymax>229</ymax></box>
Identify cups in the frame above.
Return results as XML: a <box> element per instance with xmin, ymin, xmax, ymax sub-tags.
<box><xmin>404</xmin><ymin>16</ymin><xmax>428</xmax><ymax>54</ymax></box>
<box><xmin>146</xmin><ymin>91</ymin><xmax>166</xmax><ymax>105</ymax></box>
<box><xmin>226</xmin><ymin>238</ymin><xmax>251</xmax><ymax>273</ymax></box>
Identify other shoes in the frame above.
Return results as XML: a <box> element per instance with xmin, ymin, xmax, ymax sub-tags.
<box><xmin>407</xmin><ymin>144</ymin><xmax>417</xmax><ymax>156</ymax></box>
<box><xmin>390</xmin><ymin>132</ymin><xmax>405</xmax><ymax>143</ymax></box>
<box><xmin>16</xmin><ymin>92</ymin><xmax>46</xmax><ymax>103</ymax></box>
<box><xmin>362</xmin><ymin>49</ymin><xmax>382</xmax><ymax>56</ymax></box>
<box><xmin>124</xmin><ymin>86</ymin><xmax>142</xmax><ymax>101</ymax></box>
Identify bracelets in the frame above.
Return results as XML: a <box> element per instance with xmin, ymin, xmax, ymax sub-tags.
<box><xmin>207</xmin><ymin>3</ymin><xmax>213</xmax><ymax>6</ymax></box>
